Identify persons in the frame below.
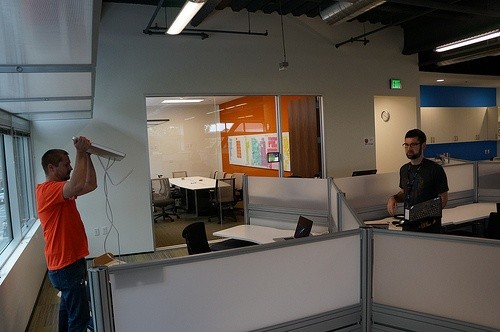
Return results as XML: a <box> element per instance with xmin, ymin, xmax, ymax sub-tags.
<box><xmin>387</xmin><ymin>128</ymin><xmax>449</xmax><ymax>233</ymax></box>
<box><xmin>36</xmin><ymin>136</ymin><xmax>97</xmax><ymax>332</ymax></box>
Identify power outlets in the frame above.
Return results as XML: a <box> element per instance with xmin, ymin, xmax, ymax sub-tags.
<box><xmin>94</xmin><ymin>226</ymin><xmax>107</xmax><ymax>235</ymax></box>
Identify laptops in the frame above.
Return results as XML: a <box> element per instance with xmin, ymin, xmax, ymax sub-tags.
<box><xmin>273</xmin><ymin>215</ymin><xmax>313</xmax><ymax>242</ymax></box>
<box><xmin>396</xmin><ymin>196</ymin><xmax>444</xmax><ymax>228</ymax></box>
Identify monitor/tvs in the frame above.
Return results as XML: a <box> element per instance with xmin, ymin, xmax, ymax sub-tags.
<box><xmin>267</xmin><ymin>152</ymin><xmax>279</xmax><ymax>163</ymax></box>
<box><xmin>71</xmin><ymin>136</ymin><xmax>127</xmax><ymax>162</ymax></box>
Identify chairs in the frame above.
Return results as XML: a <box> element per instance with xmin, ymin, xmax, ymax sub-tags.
<box><xmin>181</xmin><ymin>222</ymin><xmax>210</xmax><ymax>254</ymax></box>
<box><xmin>151</xmin><ymin>169</ymin><xmax>243</xmax><ymax>224</ymax></box>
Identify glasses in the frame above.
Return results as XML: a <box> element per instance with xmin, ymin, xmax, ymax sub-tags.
<box><xmin>402</xmin><ymin>143</ymin><xmax>420</xmax><ymax>148</ymax></box>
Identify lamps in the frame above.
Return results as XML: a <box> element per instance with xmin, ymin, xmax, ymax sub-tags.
<box><xmin>278</xmin><ymin>15</ymin><xmax>289</xmax><ymax>71</ymax></box>
<box><xmin>432</xmin><ymin>28</ymin><xmax>500</xmax><ymax>53</ymax></box>
<box><xmin>165</xmin><ymin>0</ymin><xmax>208</xmax><ymax>36</ymax></box>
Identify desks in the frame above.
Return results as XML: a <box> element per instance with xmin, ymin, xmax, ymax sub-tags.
<box><xmin>366</xmin><ymin>202</ymin><xmax>497</xmax><ymax>231</ymax></box>
<box><xmin>212</xmin><ymin>225</ymin><xmax>321</xmax><ymax>244</ymax></box>
<box><xmin>169</xmin><ymin>176</ymin><xmax>231</xmax><ymax>224</ymax></box>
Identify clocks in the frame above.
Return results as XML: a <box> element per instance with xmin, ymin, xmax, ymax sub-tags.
<box><xmin>381</xmin><ymin>111</ymin><xmax>390</xmax><ymax>122</ymax></box>
